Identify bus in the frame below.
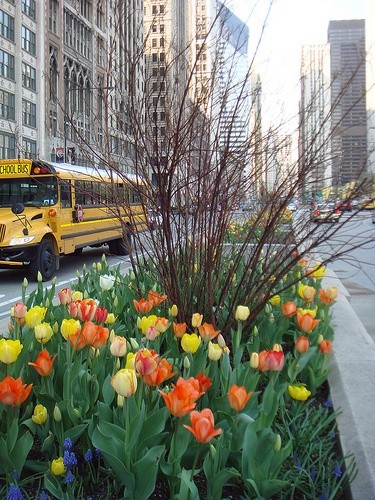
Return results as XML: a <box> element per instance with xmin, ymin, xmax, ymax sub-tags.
<box><xmin>0</xmin><ymin>158</ymin><xmax>151</xmax><ymax>282</ymax></box>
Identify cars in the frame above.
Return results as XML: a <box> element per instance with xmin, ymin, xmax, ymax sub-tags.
<box><xmin>310</xmin><ymin>203</ymin><xmax>341</xmax><ymax>223</ymax></box>
<box><xmin>147</xmin><ymin>209</ymin><xmax>164</xmax><ymax>231</ymax></box>
<box><xmin>182</xmin><ymin>199</ymin><xmax>252</xmax><ymax>215</ymax></box>
<box><xmin>286</xmin><ymin>197</ymin><xmax>375</xmax><ymax>212</ymax></box>
